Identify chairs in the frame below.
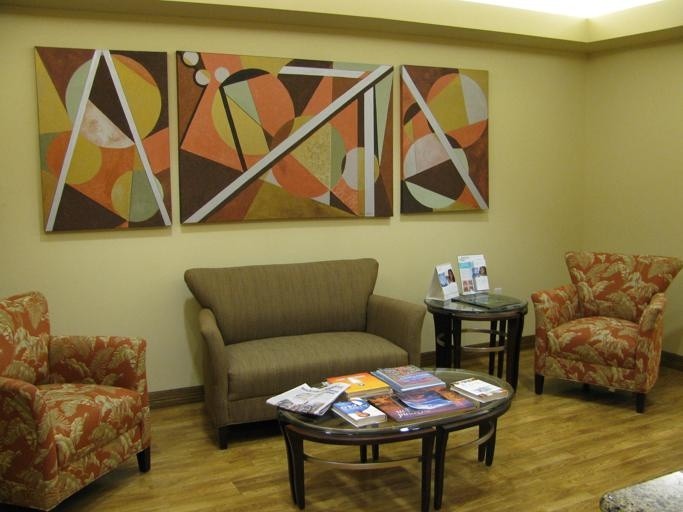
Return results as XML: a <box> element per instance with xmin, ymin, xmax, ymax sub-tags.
<box><xmin>0</xmin><ymin>288</ymin><xmax>154</xmax><ymax>512</ymax></box>
<box><xmin>529</xmin><ymin>251</ymin><xmax>682</xmax><ymax>413</ymax></box>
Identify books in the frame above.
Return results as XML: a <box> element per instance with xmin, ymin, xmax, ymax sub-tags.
<box><xmin>321</xmin><ymin>365</ymin><xmax>508</xmax><ymax>428</ymax></box>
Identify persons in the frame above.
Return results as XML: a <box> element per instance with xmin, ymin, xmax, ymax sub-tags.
<box><xmin>448</xmin><ymin>269</ymin><xmax>455</xmax><ymax>282</ymax></box>
<box><xmin>479</xmin><ymin>266</ymin><xmax>487</xmax><ymax>276</ymax></box>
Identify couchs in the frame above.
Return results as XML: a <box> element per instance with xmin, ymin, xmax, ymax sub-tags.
<box><xmin>177</xmin><ymin>258</ymin><xmax>427</xmax><ymax>450</ymax></box>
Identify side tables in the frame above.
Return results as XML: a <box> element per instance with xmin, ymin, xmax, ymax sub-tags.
<box><xmin>424</xmin><ymin>294</ymin><xmax>528</xmax><ymax>394</ymax></box>
